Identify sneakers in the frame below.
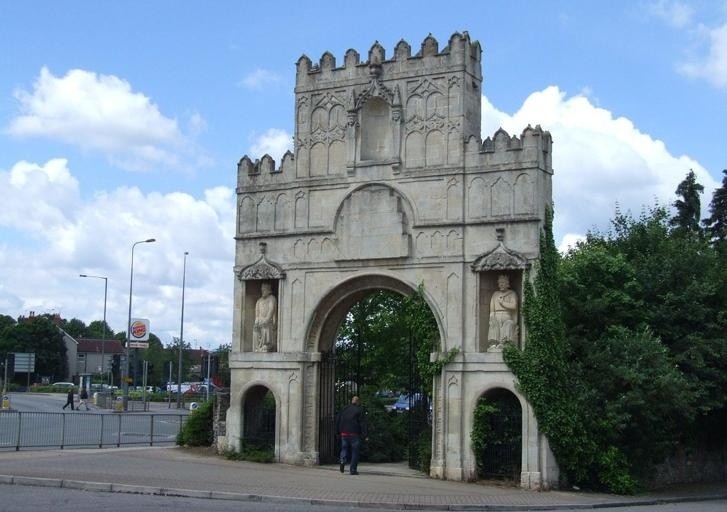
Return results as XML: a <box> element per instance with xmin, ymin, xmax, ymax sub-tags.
<box><xmin>71</xmin><ymin>408</ymin><xmax>90</xmax><ymax>410</ymax></box>
<box><xmin>62</xmin><ymin>406</ymin><xmax>65</xmax><ymax>410</ymax></box>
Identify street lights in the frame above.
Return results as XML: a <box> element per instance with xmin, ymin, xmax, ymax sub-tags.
<box><xmin>80</xmin><ymin>275</ymin><xmax>107</xmax><ymax>391</ymax></box>
<box><xmin>123</xmin><ymin>239</ymin><xmax>155</xmax><ymax>410</ymax></box>
<box><xmin>177</xmin><ymin>252</ymin><xmax>188</xmax><ymax>408</ymax></box>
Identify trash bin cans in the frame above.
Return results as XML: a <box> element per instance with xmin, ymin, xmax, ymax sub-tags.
<box><xmin>94</xmin><ymin>392</ymin><xmax>106</xmax><ymax>407</ymax></box>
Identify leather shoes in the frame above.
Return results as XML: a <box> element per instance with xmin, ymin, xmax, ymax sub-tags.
<box><xmin>340</xmin><ymin>464</ymin><xmax>359</xmax><ymax>475</ymax></box>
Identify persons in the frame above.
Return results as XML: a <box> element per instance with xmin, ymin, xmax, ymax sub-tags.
<box><xmin>76</xmin><ymin>387</ymin><xmax>90</xmax><ymax>411</ymax></box>
<box><xmin>250</xmin><ymin>283</ymin><xmax>277</xmax><ymax>354</ymax></box>
<box><xmin>483</xmin><ymin>274</ymin><xmax>522</xmax><ymax>352</ymax></box>
<box><xmin>333</xmin><ymin>394</ymin><xmax>368</xmax><ymax>476</ymax></box>
<box><xmin>62</xmin><ymin>387</ymin><xmax>76</xmax><ymax>410</ymax></box>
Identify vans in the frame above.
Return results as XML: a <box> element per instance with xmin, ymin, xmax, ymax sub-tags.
<box><xmin>52</xmin><ymin>382</ymin><xmax>75</xmax><ymax>387</ymax></box>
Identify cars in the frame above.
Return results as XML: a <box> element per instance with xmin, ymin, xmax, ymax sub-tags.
<box><xmin>392</xmin><ymin>393</ymin><xmax>432</xmax><ymax>414</ymax></box>
<box><xmin>376</xmin><ymin>390</ymin><xmax>392</xmax><ymax>398</ymax></box>
<box><xmin>92</xmin><ymin>384</ymin><xmax>161</xmax><ymax>394</ymax></box>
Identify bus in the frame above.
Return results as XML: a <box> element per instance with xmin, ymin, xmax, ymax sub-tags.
<box><xmin>167</xmin><ymin>385</ymin><xmax>208</xmax><ymax>394</ymax></box>
